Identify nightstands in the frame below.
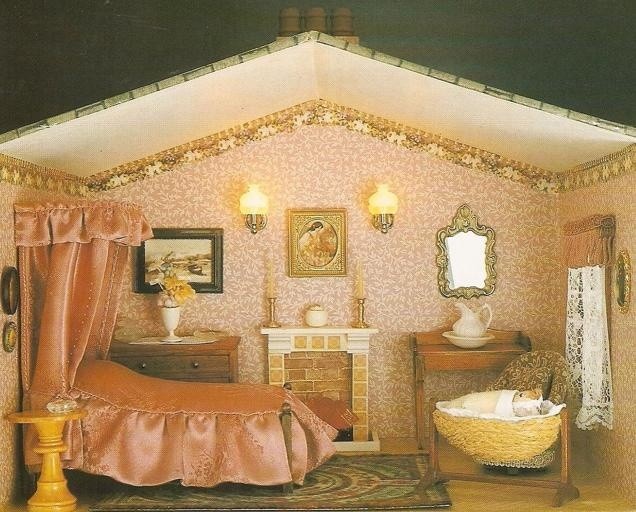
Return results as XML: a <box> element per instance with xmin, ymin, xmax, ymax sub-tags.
<box><xmin>109</xmin><ymin>335</ymin><xmax>242</xmax><ymax>384</ymax></box>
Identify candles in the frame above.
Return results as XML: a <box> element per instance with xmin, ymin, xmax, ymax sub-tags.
<box><xmin>264</xmin><ymin>258</ymin><xmax>274</xmax><ymax>300</ymax></box>
<box><xmin>355</xmin><ymin>258</ymin><xmax>365</xmax><ymax>300</ymax></box>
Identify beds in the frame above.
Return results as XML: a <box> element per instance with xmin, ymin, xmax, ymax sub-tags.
<box><xmin>15</xmin><ymin>203</ymin><xmax>342</xmax><ymax>489</ymax></box>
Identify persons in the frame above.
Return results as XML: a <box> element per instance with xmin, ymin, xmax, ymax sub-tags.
<box><xmin>298</xmin><ymin>222</ymin><xmax>333</xmax><ymax>267</ymax></box>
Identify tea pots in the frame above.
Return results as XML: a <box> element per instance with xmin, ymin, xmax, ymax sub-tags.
<box><xmin>452</xmin><ymin>302</ymin><xmax>492</xmax><ymax>337</ymax></box>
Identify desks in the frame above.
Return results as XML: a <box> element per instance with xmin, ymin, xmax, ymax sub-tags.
<box><xmin>410</xmin><ymin>325</ymin><xmax>531</xmax><ymax>454</ymax></box>
<box><xmin>7</xmin><ymin>405</ymin><xmax>91</xmax><ymax>511</ymax></box>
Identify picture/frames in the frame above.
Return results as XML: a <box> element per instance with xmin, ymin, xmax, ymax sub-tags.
<box><xmin>284</xmin><ymin>207</ymin><xmax>346</xmax><ymax>278</ymax></box>
<box><xmin>134</xmin><ymin>229</ymin><xmax>223</xmax><ymax>294</ymax></box>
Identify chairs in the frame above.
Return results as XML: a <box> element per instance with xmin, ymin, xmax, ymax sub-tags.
<box><xmin>475</xmin><ymin>348</ymin><xmax>570</xmax><ymax>474</ymax></box>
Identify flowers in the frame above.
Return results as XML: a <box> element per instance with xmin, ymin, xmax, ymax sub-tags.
<box><xmin>146</xmin><ymin>250</ymin><xmax>195</xmax><ymax>308</ymax></box>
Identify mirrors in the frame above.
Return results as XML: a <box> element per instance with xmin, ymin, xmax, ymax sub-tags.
<box><xmin>435</xmin><ymin>201</ymin><xmax>497</xmax><ymax>300</ymax></box>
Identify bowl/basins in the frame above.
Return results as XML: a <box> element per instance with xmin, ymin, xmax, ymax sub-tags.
<box><xmin>46</xmin><ymin>399</ymin><xmax>78</xmax><ymax>414</ymax></box>
<box><xmin>442</xmin><ymin>332</ymin><xmax>494</xmax><ymax>349</ymax></box>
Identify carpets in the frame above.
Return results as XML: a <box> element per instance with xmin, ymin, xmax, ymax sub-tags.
<box><xmin>88</xmin><ymin>452</ymin><xmax>453</xmax><ymax>512</ymax></box>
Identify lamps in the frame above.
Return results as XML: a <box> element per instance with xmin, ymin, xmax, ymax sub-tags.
<box><xmin>367</xmin><ymin>182</ymin><xmax>399</xmax><ymax>233</ymax></box>
<box><xmin>239</xmin><ymin>182</ymin><xmax>269</xmax><ymax>233</ymax></box>
